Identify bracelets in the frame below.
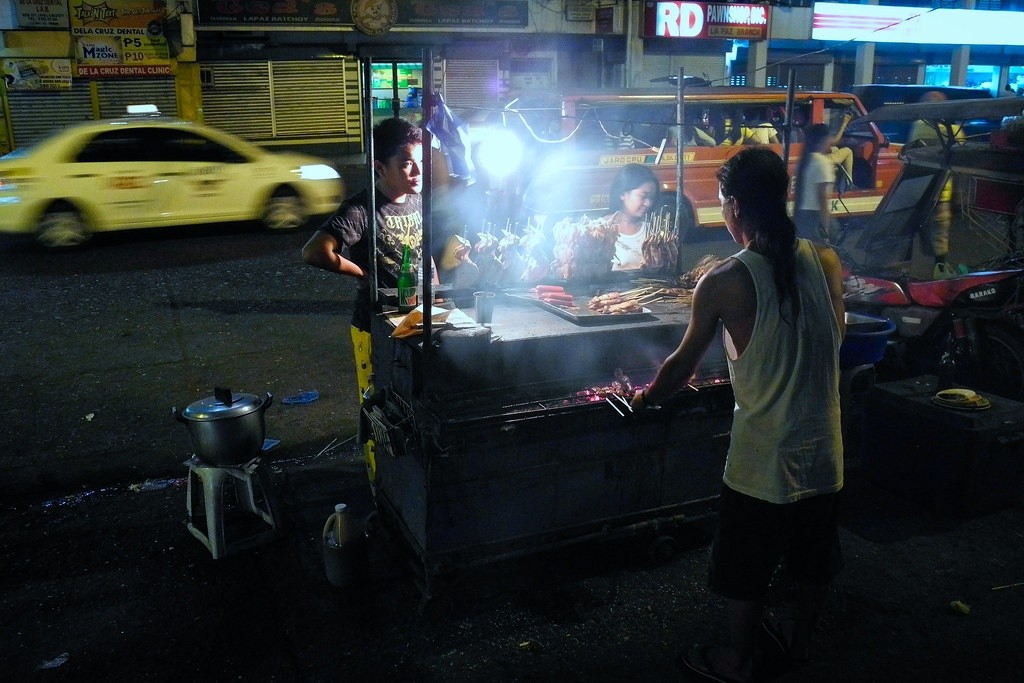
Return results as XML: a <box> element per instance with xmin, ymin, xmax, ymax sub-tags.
<box><xmin>642</xmin><ymin>390</ymin><xmax>648</xmax><ymax>404</ymax></box>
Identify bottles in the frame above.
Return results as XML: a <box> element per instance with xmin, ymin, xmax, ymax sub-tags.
<box><xmin>322</xmin><ymin>504</ymin><xmax>358</xmax><ymax>586</ymax></box>
<box><xmin>398</xmin><ymin>243</ymin><xmax>419</xmax><ymax>314</ymax></box>
<box><xmin>418</xmin><ymin>253</ymin><xmax>435</xmax><ymax>305</ymax></box>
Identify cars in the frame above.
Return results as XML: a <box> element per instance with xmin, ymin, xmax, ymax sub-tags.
<box><xmin>0</xmin><ymin>104</ymin><xmax>346</xmax><ymax>252</ymax></box>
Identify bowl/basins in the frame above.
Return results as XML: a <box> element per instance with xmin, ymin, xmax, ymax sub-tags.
<box><xmin>840</xmin><ymin>311</ymin><xmax>896</xmax><ymax>366</ymax></box>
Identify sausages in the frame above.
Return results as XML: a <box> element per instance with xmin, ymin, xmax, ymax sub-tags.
<box><xmin>536</xmin><ymin>285</ymin><xmax>574</xmax><ymax>306</ymax></box>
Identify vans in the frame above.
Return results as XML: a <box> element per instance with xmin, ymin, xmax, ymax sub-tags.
<box><xmin>558</xmin><ymin>90</ymin><xmax>911</xmax><ymax>242</ymax></box>
<box><xmin>852</xmin><ymin>83</ymin><xmax>1003</xmax><ymax>144</ymax></box>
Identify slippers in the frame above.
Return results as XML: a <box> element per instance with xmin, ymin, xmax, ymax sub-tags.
<box><xmin>678</xmin><ymin>643</ymin><xmax>739</xmax><ymax>683</ymax></box>
<box><xmin>762</xmin><ymin>617</ymin><xmax>814</xmax><ymax>666</ymax></box>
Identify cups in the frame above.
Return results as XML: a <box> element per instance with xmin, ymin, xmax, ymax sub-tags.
<box><xmin>473</xmin><ymin>291</ymin><xmax>496</xmax><ymax>323</ymax></box>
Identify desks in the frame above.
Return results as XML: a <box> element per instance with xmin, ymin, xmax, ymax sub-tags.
<box><xmin>870</xmin><ymin>375</ymin><xmax>1024</xmax><ymax>524</ymax></box>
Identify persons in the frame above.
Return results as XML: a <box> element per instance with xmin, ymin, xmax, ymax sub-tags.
<box><xmin>601</xmin><ymin>163</ymin><xmax>660</xmax><ymax>271</ymax></box>
<box><xmin>793</xmin><ymin>124</ymin><xmax>836</xmax><ymax>246</ymax></box>
<box><xmin>1004</xmin><ymin>84</ymin><xmax>1024</xmax><ymax>97</ymax></box>
<box><xmin>579</xmin><ymin>107</ymin><xmax>853</xmax><ymax>196</ymax></box>
<box><xmin>909</xmin><ymin>92</ymin><xmax>966</xmax><ymax>272</ymax></box>
<box><xmin>630</xmin><ymin>148</ymin><xmax>848</xmax><ymax>675</ymax></box>
<box><xmin>300</xmin><ymin>118</ymin><xmax>439</xmax><ymax>511</ymax></box>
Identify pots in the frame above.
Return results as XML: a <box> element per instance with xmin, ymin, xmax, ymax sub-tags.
<box><xmin>172</xmin><ymin>386</ymin><xmax>273</xmax><ymax>466</ymax></box>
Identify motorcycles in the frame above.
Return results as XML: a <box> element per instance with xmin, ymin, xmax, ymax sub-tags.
<box><xmin>837</xmin><ymin>260</ymin><xmax>1023</xmax><ymax>402</ymax></box>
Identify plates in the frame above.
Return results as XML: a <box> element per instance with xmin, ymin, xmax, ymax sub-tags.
<box><xmin>931</xmin><ymin>389</ymin><xmax>991</xmax><ymax>410</ymax></box>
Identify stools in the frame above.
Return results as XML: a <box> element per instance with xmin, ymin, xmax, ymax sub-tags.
<box><xmin>184</xmin><ymin>460</ymin><xmax>289</xmax><ymax>559</ymax></box>
